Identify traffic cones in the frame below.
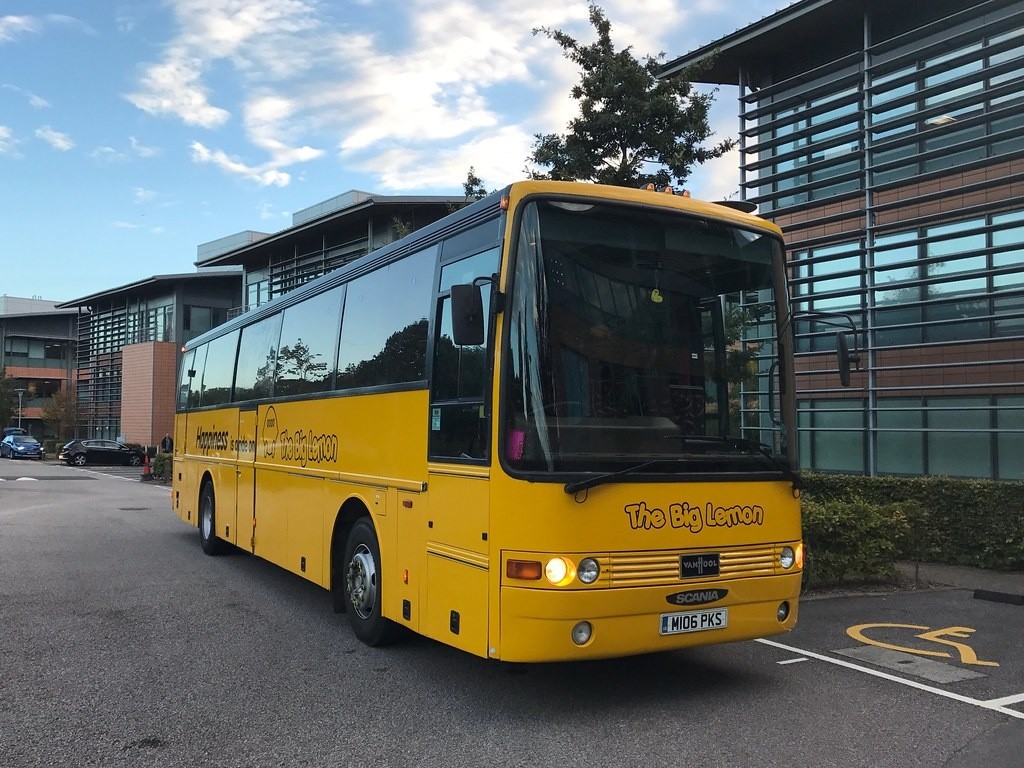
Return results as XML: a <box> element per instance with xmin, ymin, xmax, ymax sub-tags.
<box><xmin>140</xmin><ymin>455</ymin><xmax>154</xmax><ymax>481</ymax></box>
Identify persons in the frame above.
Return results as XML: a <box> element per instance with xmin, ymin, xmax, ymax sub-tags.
<box><xmin>160</xmin><ymin>433</ymin><xmax>173</xmax><ymax>453</ymax></box>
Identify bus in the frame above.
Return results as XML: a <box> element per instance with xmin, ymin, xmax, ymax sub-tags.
<box><xmin>172</xmin><ymin>180</ymin><xmax>861</xmax><ymax>664</ymax></box>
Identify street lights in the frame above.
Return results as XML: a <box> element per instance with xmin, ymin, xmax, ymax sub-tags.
<box><xmin>14</xmin><ymin>389</ymin><xmax>26</xmax><ymax>428</ymax></box>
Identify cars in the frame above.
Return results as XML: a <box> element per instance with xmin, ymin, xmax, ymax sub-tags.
<box><xmin>0</xmin><ymin>428</ymin><xmax>45</xmax><ymax>460</ymax></box>
<box><xmin>59</xmin><ymin>439</ymin><xmax>150</xmax><ymax>466</ymax></box>
